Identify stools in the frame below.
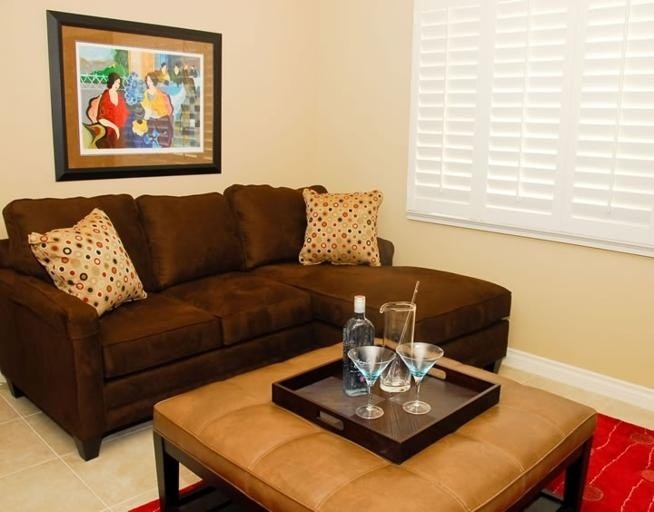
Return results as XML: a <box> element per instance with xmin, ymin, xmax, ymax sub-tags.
<box><xmin>150</xmin><ymin>341</ymin><xmax>599</xmax><ymax>512</ymax></box>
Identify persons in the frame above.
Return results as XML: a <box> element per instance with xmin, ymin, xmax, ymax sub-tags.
<box><xmin>172</xmin><ymin>63</ymin><xmax>182</xmax><ymax>78</ymax></box>
<box><xmin>189</xmin><ymin>65</ymin><xmax>197</xmax><ymax>76</ymax></box>
<box><xmin>91</xmin><ymin>71</ymin><xmax>132</xmax><ymax>148</ymax></box>
<box><xmin>159</xmin><ymin>62</ymin><xmax>171</xmax><ymax>81</ymax></box>
<box><xmin>182</xmin><ymin>64</ymin><xmax>189</xmax><ymax>76</ymax></box>
<box><xmin>138</xmin><ymin>71</ymin><xmax>175</xmax><ymax>149</ymax></box>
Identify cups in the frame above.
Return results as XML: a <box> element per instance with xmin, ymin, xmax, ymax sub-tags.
<box><xmin>378</xmin><ymin>303</ymin><xmax>416</xmax><ymax>393</ymax></box>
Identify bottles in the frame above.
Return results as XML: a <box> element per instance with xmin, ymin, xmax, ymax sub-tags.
<box><xmin>344</xmin><ymin>296</ymin><xmax>378</xmax><ymax>398</ymax></box>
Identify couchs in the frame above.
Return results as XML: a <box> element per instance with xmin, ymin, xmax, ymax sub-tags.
<box><xmin>0</xmin><ymin>240</ymin><xmax>511</xmax><ymax>462</ymax></box>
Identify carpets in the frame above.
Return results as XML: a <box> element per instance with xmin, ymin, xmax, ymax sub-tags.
<box><xmin>129</xmin><ymin>415</ymin><xmax>652</xmax><ymax>512</ymax></box>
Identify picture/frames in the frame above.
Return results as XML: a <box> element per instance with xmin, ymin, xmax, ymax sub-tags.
<box><xmin>45</xmin><ymin>9</ymin><xmax>224</xmax><ymax>183</ymax></box>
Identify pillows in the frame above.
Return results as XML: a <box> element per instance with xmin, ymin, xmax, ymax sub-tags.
<box><xmin>27</xmin><ymin>207</ymin><xmax>149</xmax><ymax>318</ymax></box>
<box><xmin>1</xmin><ymin>193</ymin><xmax>157</xmax><ymax>302</ymax></box>
<box><xmin>225</xmin><ymin>184</ymin><xmax>329</xmax><ymax>272</ymax></box>
<box><xmin>297</xmin><ymin>188</ymin><xmax>384</xmax><ymax>268</ymax></box>
<box><xmin>134</xmin><ymin>192</ymin><xmax>247</xmax><ymax>292</ymax></box>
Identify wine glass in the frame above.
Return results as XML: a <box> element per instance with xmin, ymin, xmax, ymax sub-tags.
<box><xmin>347</xmin><ymin>342</ymin><xmax>443</xmax><ymax>420</ymax></box>
